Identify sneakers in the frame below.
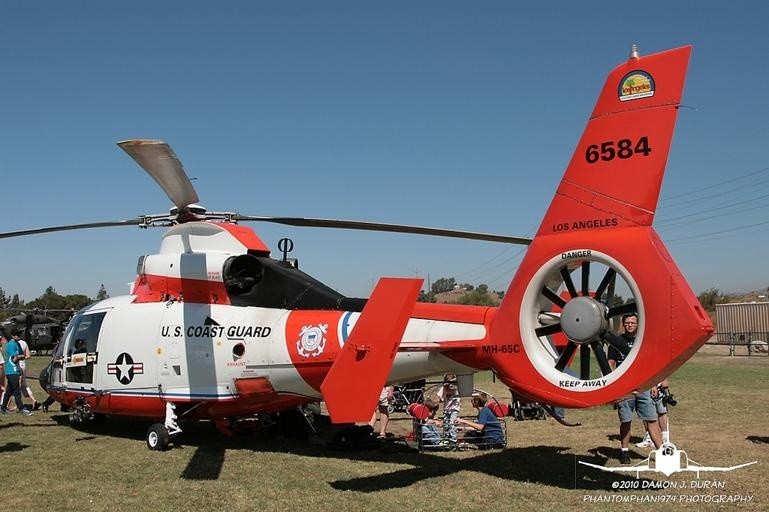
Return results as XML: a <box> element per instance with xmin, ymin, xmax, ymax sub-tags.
<box><xmin>620</xmin><ymin>450</ymin><xmax>631</xmax><ymax>464</ymax></box>
<box><xmin>636</xmin><ymin>439</ymin><xmax>655</xmax><ymax>448</ymax></box>
<box><xmin>459</xmin><ymin>442</ymin><xmax>479</xmax><ymax>451</ymax></box>
<box><xmin>0</xmin><ymin>400</ymin><xmax>49</xmax><ymax>416</ymax></box>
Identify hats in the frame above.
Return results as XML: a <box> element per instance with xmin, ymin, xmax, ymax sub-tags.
<box><xmin>10</xmin><ymin>328</ymin><xmax>22</xmax><ymax>336</ymax></box>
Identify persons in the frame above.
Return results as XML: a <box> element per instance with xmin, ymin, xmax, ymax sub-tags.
<box><xmin>70</xmin><ymin>338</ymin><xmax>92</xmax><ymax>381</ymax></box>
<box><xmin>432</xmin><ymin>370</ymin><xmax>462</xmax><ymax>446</ymax></box>
<box><xmin>8</xmin><ymin>327</ymin><xmax>42</xmax><ymax>412</ymax></box>
<box><xmin>413</xmin><ymin>398</ymin><xmax>451</xmax><ymax>451</ymax></box>
<box><xmin>453</xmin><ymin>391</ymin><xmax>506</xmax><ymax>451</ymax></box>
<box><xmin>0</xmin><ymin>334</ymin><xmax>9</xmax><ymax>405</ymax></box>
<box><xmin>602</xmin><ymin>309</ymin><xmax>664</xmax><ymax>467</ymax></box>
<box><xmin>547</xmin><ymin>404</ymin><xmax>566</xmax><ymax>421</ymax></box>
<box><xmin>367</xmin><ymin>382</ymin><xmax>396</xmax><ymax>440</ymax></box>
<box><xmin>0</xmin><ymin>327</ymin><xmax>34</xmax><ymax>416</ymax></box>
<box><xmin>636</xmin><ymin>377</ymin><xmax>672</xmax><ymax>449</ymax></box>
<box><xmin>42</xmin><ymin>394</ymin><xmax>69</xmax><ymax>413</ymax></box>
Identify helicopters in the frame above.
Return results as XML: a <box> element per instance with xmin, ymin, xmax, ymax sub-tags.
<box><xmin>0</xmin><ymin>42</ymin><xmax>715</xmax><ymax>449</ymax></box>
<box><xmin>0</xmin><ymin>306</ymin><xmax>78</xmax><ymax>357</ymax></box>
